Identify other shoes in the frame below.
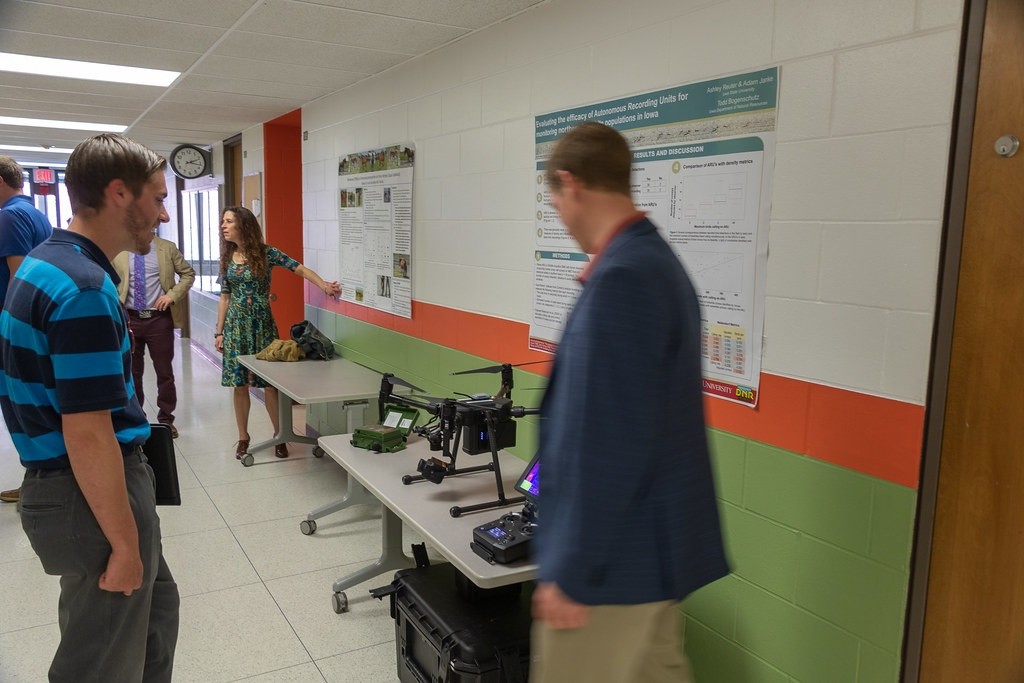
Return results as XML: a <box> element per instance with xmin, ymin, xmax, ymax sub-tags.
<box><xmin>166</xmin><ymin>422</ymin><xmax>178</xmax><ymax>439</ymax></box>
<box><xmin>0</xmin><ymin>488</ymin><xmax>21</xmax><ymax>501</ymax></box>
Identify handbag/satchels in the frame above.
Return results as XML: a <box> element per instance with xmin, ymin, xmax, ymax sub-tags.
<box><xmin>141</xmin><ymin>423</ymin><xmax>180</xmax><ymax>505</ymax></box>
<box><xmin>290</xmin><ymin>320</ymin><xmax>334</xmax><ymax>361</ymax></box>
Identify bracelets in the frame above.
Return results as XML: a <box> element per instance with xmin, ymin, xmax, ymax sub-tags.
<box><xmin>215</xmin><ymin>334</ymin><xmax>223</xmax><ymax>338</ymax></box>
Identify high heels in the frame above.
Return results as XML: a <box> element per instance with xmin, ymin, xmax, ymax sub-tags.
<box><xmin>273</xmin><ymin>433</ymin><xmax>288</xmax><ymax>457</ymax></box>
<box><xmin>233</xmin><ymin>433</ymin><xmax>250</xmax><ymax>459</ymax></box>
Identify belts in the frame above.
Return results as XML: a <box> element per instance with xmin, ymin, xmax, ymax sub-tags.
<box><xmin>25</xmin><ymin>445</ymin><xmax>144</xmax><ymax>478</ymax></box>
<box><xmin>127</xmin><ymin>308</ymin><xmax>171</xmax><ymax>319</ymax></box>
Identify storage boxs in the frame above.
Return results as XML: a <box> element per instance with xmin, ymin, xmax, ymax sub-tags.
<box><xmin>368</xmin><ymin>542</ymin><xmax>534</xmax><ymax>683</ymax></box>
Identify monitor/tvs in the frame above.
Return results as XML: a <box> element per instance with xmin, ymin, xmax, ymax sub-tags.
<box><xmin>514</xmin><ymin>448</ymin><xmax>540</xmax><ymax>501</ymax></box>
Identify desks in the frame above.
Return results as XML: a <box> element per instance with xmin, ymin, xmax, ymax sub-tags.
<box><xmin>301</xmin><ymin>423</ymin><xmax>541</xmax><ymax>614</ymax></box>
<box><xmin>235</xmin><ymin>352</ymin><xmax>414</xmax><ymax>536</ymax></box>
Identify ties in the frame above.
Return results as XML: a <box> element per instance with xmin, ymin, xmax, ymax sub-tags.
<box><xmin>134</xmin><ymin>253</ymin><xmax>147</xmax><ymax>312</ymax></box>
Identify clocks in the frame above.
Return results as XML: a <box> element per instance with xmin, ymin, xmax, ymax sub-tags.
<box><xmin>170</xmin><ymin>144</ymin><xmax>214</xmax><ymax>179</ymax></box>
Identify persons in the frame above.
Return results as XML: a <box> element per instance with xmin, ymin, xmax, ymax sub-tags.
<box><xmin>0</xmin><ymin>134</ymin><xmax>180</xmax><ymax>683</ymax></box>
<box><xmin>527</xmin><ymin>122</ymin><xmax>733</xmax><ymax>682</ymax></box>
<box><xmin>215</xmin><ymin>207</ymin><xmax>340</xmax><ymax>459</ymax></box>
<box><xmin>0</xmin><ymin>157</ymin><xmax>53</xmax><ymax>502</ymax></box>
<box><xmin>112</xmin><ymin>237</ymin><xmax>196</xmax><ymax>439</ymax></box>
